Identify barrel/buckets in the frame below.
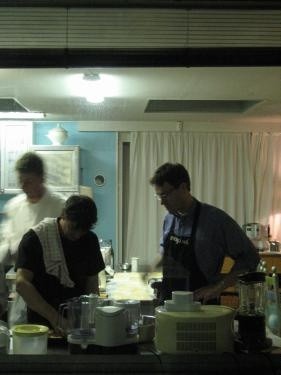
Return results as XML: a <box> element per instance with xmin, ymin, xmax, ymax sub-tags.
<box><xmin>12</xmin><ymin>324</ymin><xmax>49</xmax><ymax>355</ymax></box>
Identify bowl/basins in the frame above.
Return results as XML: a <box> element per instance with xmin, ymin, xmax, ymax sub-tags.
<box><xmin>119</xmin><ymin>263</ymin><xmax>131</xmax><ymax>270</ymax></box>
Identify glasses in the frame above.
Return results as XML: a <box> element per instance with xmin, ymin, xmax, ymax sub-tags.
<box><xmin>156</xmin><ymin>188</ymin><xmax>177</xmax><ymax>201</ymax></box>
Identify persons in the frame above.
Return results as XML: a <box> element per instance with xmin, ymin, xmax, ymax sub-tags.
<box><xmin>15</xmin><ymin>195</ymin><xmax>106</xmax><ymax>340</ymax></box>
<box><xmin>0</xmin><ymin>152</ymin><xmax>67</xmax><ymax>328</ymax></box>
<box><xmin>146</xmin><ymin>161</ymin><xmax>262</xmax><ymax>313</ymax></box>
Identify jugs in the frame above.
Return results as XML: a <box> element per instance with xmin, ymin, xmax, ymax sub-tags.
<box><xmin>56</xmin><ymin>296</ymin><xmax>97</xmax><ymax>340</ymax></box>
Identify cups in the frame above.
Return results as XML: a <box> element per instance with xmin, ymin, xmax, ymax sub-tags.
<box><xmin>130</xmin><ymin>258</ymin><xmax>139</xmax><ymax>272</ymax></box>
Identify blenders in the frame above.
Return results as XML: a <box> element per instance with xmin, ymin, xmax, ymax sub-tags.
<box><xmin>234</xmin><ymin>271</ymin><xmax>273</xmax><ymax>354</ymax></box>
